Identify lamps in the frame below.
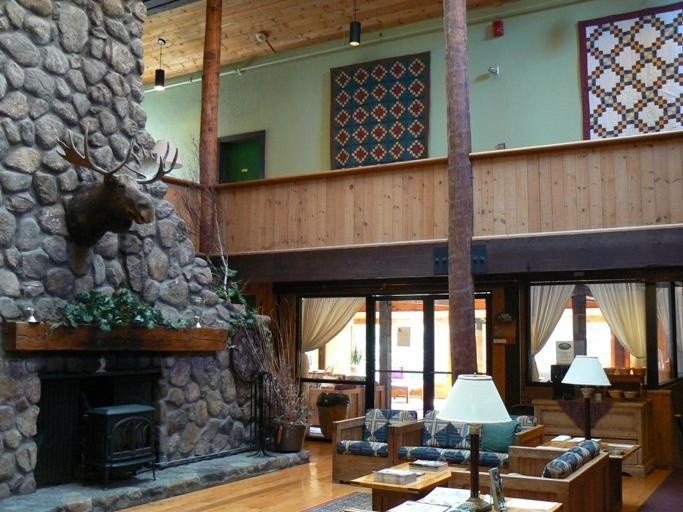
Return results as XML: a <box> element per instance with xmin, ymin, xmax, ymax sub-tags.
<box><xmin>559</xmin><ymin>352</ymin><xmax>612</xmax><ymax>443</ymax></box>
<box><xmin>349</xmin><ymin>0</ymin><xmax>360</xmax><ymax>47</ymax></box>
<box><xmin>435</xmin><ymin>375</ymin><xmax>513</xmax><ymax>512</ymax></box>
<box><xmin>154</xmin><ymin>39</ymin><xmax>165</xmax><ymax>91</ymax></box>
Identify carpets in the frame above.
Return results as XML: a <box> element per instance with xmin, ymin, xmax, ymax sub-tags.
<box><xmin>300</xmin><ymin>491</ymin><xmax>372</xmax><ymax>512</ymax></box>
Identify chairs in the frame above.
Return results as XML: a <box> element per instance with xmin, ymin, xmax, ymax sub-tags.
<box><xmin>333</xmin><ymin>409</ymin><xmax>423</xmax><ymax>484</ymax></box>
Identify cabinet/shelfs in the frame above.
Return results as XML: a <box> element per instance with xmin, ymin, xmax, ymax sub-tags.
<box><xmin>531</xmin><ymin>398</ymin><xmax>655</xmax><ymax>478</ymax></box>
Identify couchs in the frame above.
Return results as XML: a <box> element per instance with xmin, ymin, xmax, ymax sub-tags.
<box><xmin>450</xmin><ymin>445</ymin><xmax>610</xmax><ymax>512</ymax></box>
<box><xmin>393</xmin><ymin>409</ymin><xmax>545</xmax><ymax>484</ymax></box>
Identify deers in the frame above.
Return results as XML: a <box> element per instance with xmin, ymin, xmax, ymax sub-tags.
<box><xmin>55</xmin><ymin>122</ymin><xmax>179</xmax><ymax>249</ymax></box>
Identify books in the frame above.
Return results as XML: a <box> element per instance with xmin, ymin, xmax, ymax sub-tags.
<box><xmin>372</xmin><ymin>459</ymin><xmax>448</xmax><ymax>486</ymax></box>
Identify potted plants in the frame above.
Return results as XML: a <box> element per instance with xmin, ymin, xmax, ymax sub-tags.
<box><xmin>316</xmin><ymin>391</ymin><xmax>350</xmax><ymax>440</ymax></box>
<box><xmin>237</xmin><ymin>305</ymin><xmax>312</xmax><ymax>453</ymax></box>
<box><xmin>608</xmin><ymin>381</ymin><xmax>639</xmax><ymax>400</ymax></box>
